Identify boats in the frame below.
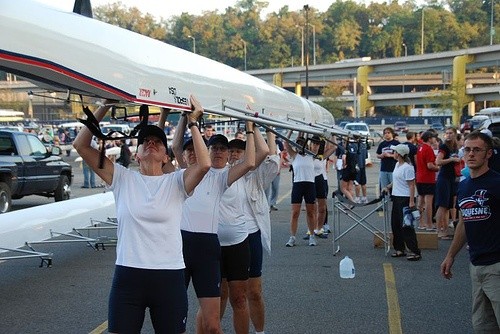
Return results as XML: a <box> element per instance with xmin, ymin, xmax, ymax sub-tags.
<box><xmin>0</xmin><ymin>0</ymin><xmax>335</xmax><ymax>137</ymax></box>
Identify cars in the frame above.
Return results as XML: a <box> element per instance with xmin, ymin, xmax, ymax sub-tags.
<box><xmin>338</xmin><ymin>121</ymin><xmax>351</xmax><ymax>129</ymax></box>
<box><xmin>430</xmin><ymin>122</ymin><xmax>444</xmax><ymax>131</ymax></box>
<box><xmin>394</xmin><ymin>121</ymin><xmax>410</xmax><ymax>134</ymax></box>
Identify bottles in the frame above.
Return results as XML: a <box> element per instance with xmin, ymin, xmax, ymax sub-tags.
<box><xmin>339</xmin><ymin>256</ymin><xmax>355</xmax><ymax>279</ymax></box>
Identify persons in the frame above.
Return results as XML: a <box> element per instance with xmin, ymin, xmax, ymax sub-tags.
<box><xmin>284</xmin><ymin>130</ymin><xmax>337</xmax><ymax>247</ymax></box>
<box><xmin>81</xmin><ymin>140</ymin><xmax>98</xmax><ymax>188</ymax></box>
<box><xmin>172</xmin><ymin>110</ymin><xmax>277</xmax><ymax>334</ymax></box>
<box><xmin>381</xmin><ymin>144</ymin><xmax>421</xmax><ymax>261</ymax></box>
<box><xmin>375</xmin><ymin>115</ymin><xmax>500</xmax><ymax>240</ymax></box>
<box><xmin>116</xmin><ymin>138</ymin><xmax>133</xmax><ymax>168</ymax></box>
<box><xmin>23</xmin><ymin>121</ymin><xmax>202</xmax><ymax>157</ymax></box>
<box><xmin>157</xmin><ymin>106</ymin><xmax>257</xmax><ymax>334</ymax></box>
<box><xmin>74</xmin><ymin>94</ymin><xmax>212</xmax><ymax>334</ymax></box>
<box><xmin>202</xmin><ymin>125</ymin><xmax>217</xmax><ymax>149</ymax></box>
<box><xmin>439</xmin><ymin>132</ymin><xmax>500</xmax><ymax>334</ymax></box>
<box><xmin>170</xmin><ymin>105</ymin><xmax>271</xmax><ymax>334</ymax></box>
<box><xmin>266</xmin><ymin>139</ymin><xmax>284</xmax><ymax>211</ymax></box>
<box><xmin>333</xmin><ymin>132</ymin><xmax>369</xmax><ymax>210</ymax></box>
<box><xmin>235</xmin><ymin>132</ymin><xmax>244</xmax><ymax>141</ymax></box>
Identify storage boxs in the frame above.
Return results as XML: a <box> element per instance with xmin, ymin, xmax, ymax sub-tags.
<box><xmin>374</xmin><ymin>231</ymin><xmax>438</xmax><ymax>250</ymax></box>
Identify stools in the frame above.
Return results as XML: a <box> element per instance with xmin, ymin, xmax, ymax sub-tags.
<box><xmin>332</xmin><ymin>187</ymin><xmax>390</xmax><ymax>255</ymax></box>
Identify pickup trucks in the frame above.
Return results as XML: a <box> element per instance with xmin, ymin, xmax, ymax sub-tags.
<box><xmin>0</xmin><ymin>130</ymin><xmax>74</xmax><ymax>213</ymax></box>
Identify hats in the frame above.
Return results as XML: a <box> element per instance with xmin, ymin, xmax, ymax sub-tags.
<box><xmin>183</xmin><ymin>137</ymin><xmax>209</xmax><ymax>150</ymax></box>
<box><xmin>389</xmin><ymin>144</ymin><xmax>410</xmax><ymax>156</ymax></box>
<box><xmin>228</xmin><ymin>139</ymin><xmax>246</xmax><ymax>150</ymax></box>
<box><xmin>208</xmin><ymin>134</ymin><xmax>228</xmax><ymax>146</ymax></box>
<box><xmin>137</xmin><ymin>124</ymin><xmax>168</xmax><ymax>148</ymax></box>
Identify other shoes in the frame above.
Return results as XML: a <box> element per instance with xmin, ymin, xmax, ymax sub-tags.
<box><xmin>391</xmin><ymin>251</ymin><xmax>407</xmax><ymax>258</ymax></box>
<box><xmin>418</xmin><ymin>225</ymin><xmax>438</xmax><ymax>231</ymax></box>
<box><xmin>285</xmin><ymin>237</ymin><xmax>296</xmax><ymax>247</ymax></box>
<box><xmin>438</xmin><ymin>233</ymin><xmax>454</xmax><ymax>240</ymax></box>
<box><xmin>402</xmin><ymin>253</ymin><xmax>422</xmax><ymax>261</ymax></box>
<box><xmin>303</xmin><ymin>224</ymin><xmax>331</xmax><ymax>239</ymax></box>
<box><xmin>354</xmin><ymin>196</ymin><xmax>368</xmax><ymax>203</ymax></box>
<box><xmin>309</xmin><ymin>236</ymin><xmax>318</xmax><ymax>246</ymax></box>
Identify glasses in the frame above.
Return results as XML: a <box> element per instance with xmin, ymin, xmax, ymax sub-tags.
<box><xmin>211</xmin><ymin>146</ymin><xmax>227</xmax><ymax>152</ymax></box>
<box><xmin>462</xmin><ymin>146</ymin><xmax>487</xmax><ymax>153</ymax></box>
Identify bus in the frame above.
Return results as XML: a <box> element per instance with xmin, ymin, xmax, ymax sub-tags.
<box><xmin>100</xmin><ymin>125</ymin><xmax>130</xmax><ymax>136</ymax></box>
<box><xmin>59</xmin><ymin>121</ymin><xmax>86</xmax><ymax>140</ymax></box>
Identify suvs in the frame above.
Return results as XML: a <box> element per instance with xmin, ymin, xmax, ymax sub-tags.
<box><xmin>344</xmin><ymin>123</ymin><xmax>374</xmax><ymax>149</ymax></box>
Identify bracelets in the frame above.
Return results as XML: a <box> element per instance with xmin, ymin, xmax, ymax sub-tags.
<box><xmin>246</xmin><ymin>131</ymin><xmax>255</xmax><ymax>134</ymax></box>
<box><xmin>188</xmin><ymin>121</ymin><xmax>199</xmax><ymax>129</ymax></box>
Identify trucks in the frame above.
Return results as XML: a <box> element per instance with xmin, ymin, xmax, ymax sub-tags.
<box><xmin>469</xmin><ymin>108</ymin><xmax>500</xmax><ymax>131</ymax></box>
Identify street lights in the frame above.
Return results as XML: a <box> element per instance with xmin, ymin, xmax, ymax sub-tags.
<box><xmin>239</xmin><ymin>39</ymin><xmax>247</xmax><ymax>71</ymax></box>
<box><xmin>303</xmin><ymin>23</ymin><xmax>316</xmax><ymax>66</ymax></box>
<box><xmin>401</xmin><ymin>43</ymin><xmax>407</xmax><ymax>58</ymax></box>
<box><xmin>187</xmin><ymin>35</ymin><xmax>196</xmax><ymax>54</ymax></box>
<box><xmin>303</xmin><ymin>4</ymin><xmax>310</xmax><ymax>99</ymax></box>
